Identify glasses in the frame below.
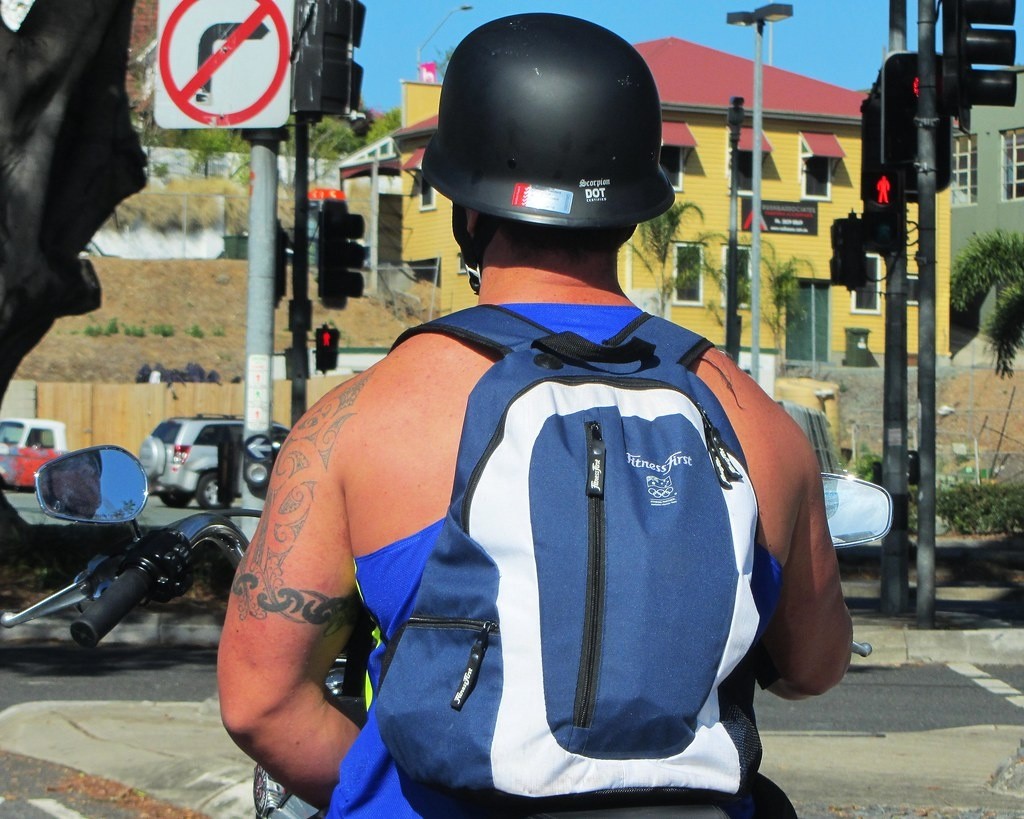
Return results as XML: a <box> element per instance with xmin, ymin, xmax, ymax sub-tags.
<box><xmin>52</xmin><ymin>454</ymin><xmax>102</xmax><ymax>475</ymax></box>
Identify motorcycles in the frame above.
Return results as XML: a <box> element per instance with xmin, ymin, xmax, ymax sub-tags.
<box><xmin>2</xmin><ymin>447</ymin><xmax>892</xmax><ymax>819</ymax></box>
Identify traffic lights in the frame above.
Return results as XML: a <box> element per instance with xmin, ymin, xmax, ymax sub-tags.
<box><xmin>860</xmin><ymin>162</ymin><xmax>905</xmax><ymax>257</ymax></box>
<box><xmin>880</xmin><ymin>48</ymin><xmax>955</xmax><ymax>198</ymax></box>
<box><xmin>314</xmin><ymin>322</ymin><xmax>340</xmax><ymax>374</ymax></box>
<box><xmin>315</xmin><ymin>196</ymin><xmax>368</xmax><ymax>312</ymax></box>
<box><xmin>826</xmin><ymin>213</ymin><xmax>869</xmax><ymax>294</ymax></box>
<box><xmin>289</xmin><ymin>0</ymin><xmax>368</xmax><ymax>127</ymax></box>
<box><xmin>941</xmin><ymin>0</ymin><xmax>1019</xmax><ymax>137</ymax></box>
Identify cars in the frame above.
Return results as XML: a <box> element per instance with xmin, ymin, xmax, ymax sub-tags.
<box><xmin>0</xmin><ymin>417</ymin><xmax>68</xmax><ymax>491</ymax></box>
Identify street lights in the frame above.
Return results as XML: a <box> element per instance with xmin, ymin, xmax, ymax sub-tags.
<box><xmin>724</xmin><ymin>3</ymin><xmax>796</xmax><ymax>393</ymax></box>
<box><xmin>416</xmin><ymin>4</ymin><xmax>473</xmax><ymax>82</ymax></box>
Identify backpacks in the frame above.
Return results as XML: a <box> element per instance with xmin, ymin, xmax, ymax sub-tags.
<box><xmin>374</xmin><ymin>305</ymin><xmax>782</xmax><ymax>815</ymax></box>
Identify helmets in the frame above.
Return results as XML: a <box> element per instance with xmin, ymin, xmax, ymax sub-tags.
<box><xmin>422</xmin><ymin>12</ymin><xmax>675</xmax><ymax>228</ymax></box>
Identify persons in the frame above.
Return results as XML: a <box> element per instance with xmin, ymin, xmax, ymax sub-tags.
<box><xmin>218</xmin><ymin>14</ymin><xmax>853</xmax><ymax>818</ymax></box>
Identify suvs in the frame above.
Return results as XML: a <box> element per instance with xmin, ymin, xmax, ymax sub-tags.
<box><xmin>139</xmin><ymin>412</ymin><xmax>291</xmax><ymax>510</ymax></box>
